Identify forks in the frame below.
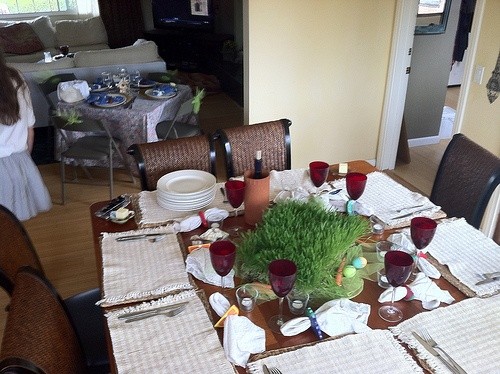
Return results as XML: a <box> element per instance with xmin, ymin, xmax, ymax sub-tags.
<box><xmin>421</xmin><ymin>328</ymin><xmax>467</xmax><ymax>374</ymax></box>
<box><xmin>270</xmin><ymin>367</ymin><xmax>282</xmax><ymax>374</ymax></box>
<box><xmin>125</xmin><ymin>304</ymin><xmax>186</xmax><ymax>322</ymax></box>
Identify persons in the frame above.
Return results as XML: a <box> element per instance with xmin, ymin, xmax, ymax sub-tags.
<box><xmin>0</xmin><ymin>51</ymin><xmax>52</xmax><ymax>227</ymax></box>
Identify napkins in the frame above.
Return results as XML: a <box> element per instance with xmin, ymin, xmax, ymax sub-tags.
<box><xmin>185</xmin><ymin>247</ymin><xmax>236</xmax><ymax>290</ymax></box>
<box><xmin>386</xmin><ymin>233</ymin><xmax>442</xmax><ymax>279</ymax></box>
<box><xmin>208</xmin><ymin>292</ymin><xmax>266</xmax><ymax>369</ymax></box>
<box><xmin>280</xmin><ymin>296</ymin><xmax>371</xmax><ymax>337</ymax></box>
<box><xmin>170</xmin><ymin>206</ymin><xmax>230</xmax><ymax>232</ymax></box>
<box><xmin>272</xmin><ymin>186</ymin><xmax>372</xmax><ymax>216</ymax></box>
<box><xmin>378</xmin><ymin>271</ymin><xmax>455</xmax><ymax>305</ymax></box>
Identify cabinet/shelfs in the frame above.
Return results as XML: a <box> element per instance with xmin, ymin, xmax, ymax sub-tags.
<box><xmin>143</xmin><ymin>30</ymin><xmax>234</xmax><ymax>80</ymax></box>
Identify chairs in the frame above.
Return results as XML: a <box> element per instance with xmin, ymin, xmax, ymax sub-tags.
<box><xmin>38</xmin><ymin>73</ymin><xmax>206</xmax><ymax>205</ymax></box>
<box><xmin>429</xmin><ymin>133</ymin><xmax>500</xmax><ymax>239</ymax></box>
<box><xmin>128</xmin><ymin>134</ymin><xmax>215</xmax><ymax>191</ymax></box>
<box><xmin>0</xmin><ymin>205</ymin><xmax>113</xmax><ymax>374</ymax></box>
<box><xmin>217</xmin><ymin>119</ymin><xmax>292</xmax><ymax>181</ymax></box>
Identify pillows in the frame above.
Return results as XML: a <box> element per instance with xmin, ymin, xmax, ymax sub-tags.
<box><xmin>0</xmin><ymin>23</ymin><xmax>44</xmax><ymax>54</ymax></box>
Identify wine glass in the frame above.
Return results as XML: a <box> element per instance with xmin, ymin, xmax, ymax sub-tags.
<box><xmin>309</xmin><ymin>160</ymin><xmax>330</xmax><ymax>196</ymax></box>
<box><xmin>378</xmin><ymin>250</ymin><xmax>414</xmax><ymax>322</ymax></box>
<box><xmin>60</xmin><ymin>46</ymin><xmax>69</xmax><ymax>57</ymax></box>
<box><xmin>408</xmin><ymin>216</ymin><xmax>438</xmax><ymax>281</ymax></box>
<box><xmin>268</xmin><ymin>258</ymin><xmax>297</xmax><ymax>335</ymax></box>
<box><xmin>101</xmin><ymin>66</ymin><xmax>142</xmax><ymax>89</ymax></box>
<box><xmin>225</xmin><ymin>180</ymin><xmax>244</xmax><ymax>237</ymax></box>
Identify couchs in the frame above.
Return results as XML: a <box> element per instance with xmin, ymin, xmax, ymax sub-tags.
<box><xmin>0</xmin><ymin>16</ymin><xmax>166</xmax><ymax>159</ymax></box>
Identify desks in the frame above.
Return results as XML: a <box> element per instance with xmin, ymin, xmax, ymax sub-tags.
<box><xmin>89</xmin><ymin>160</ymin><xmax>500</xmax><ymax>374</ymax></box>
<box><xmin>54</xmin><ymin>84</ymin><xmax>192</xmax><ymax>174</ymax></box>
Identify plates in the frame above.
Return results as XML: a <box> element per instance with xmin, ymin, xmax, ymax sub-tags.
<box><xmin>53</xmin><ymin>52</ymin><xmax>76</xmax><ymax>61</ymax></box>
<box><xmin>157</xmin><ymin>169</ymin><xmax>217</xmax><ymax>212</ymax></box>
<box><xmin>91</xmin><ymin>81</ymin><xmax>177</xmax><ymax>107</ymax></box>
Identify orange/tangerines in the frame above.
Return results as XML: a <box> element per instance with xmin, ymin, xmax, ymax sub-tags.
<box><xmin>187</xmin><ymin>243</ymin><xmax>211</xmax><ymax>254</ymax></box>
<box><xmin>213</xmin><ymin>304</ymin><xmax>239</xmax><ymax>328</ymax></box>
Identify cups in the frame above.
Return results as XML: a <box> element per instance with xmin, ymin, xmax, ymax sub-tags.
<box><xmin>236</xmin><ymin>285</ymin><xmax>259</xmax><ymax>312</ymax></box>
<box><xmin>207</xmin><ymin>213</ymin><xmax>224</xmax><ymax>230</ymax></box>
<box><xmin>376</xmin><ymin>240</ymin><xmax>395</xmax><ymax>262</ymax></box>
<box><xmin>376</xmin><ymin>263</ymin><xmax>390</xmax><ymax>289</ymax></box>
<box><xmin>243</xmin><ymin>169</ymin><xmax>270</xmax><ymax>233</ymax></box>
<box><xmin>345</xmin><ymin>171</ymin><xmax>367</xmax><ymax>201</ymax></box>
<box><xmin>369</xmin><ymin>214</ymin><xmax>386</xmax><ymax>234</ymax></box>
<box><xmin>286</xmin><ymin>289</ymin><xmax>309</xmax><ymax>315</ymax></box>
<box><xmin>44</xmin><ymin>53</ymin><xmax>52</xmax><ymax>63</ymax></box>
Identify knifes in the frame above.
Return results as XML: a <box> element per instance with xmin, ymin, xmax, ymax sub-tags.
<box><xmin>390</xmin><ymin>207</ymin><xmax>433</xmax><ymax>220</ymax></box>
<box><xmin>475</xmin><ymin>277</ymin><xmax>500</xmax><ymax>285</ymax></box>
<box><xmin>118</xmin><ymin>301</ymin><xmax>189</xmax><ymax>320</ymax></box>
<box><xmin>263</xmin><ymin>365</ymin><xmax>270</xmax><ymax>374</ymax></box>
<box><xmin>115</xmin><ymin>233</ymin><xmax>165</xmax><ymax>239</ymax></box>
<box><xmin>412</xmin><ymin>331</ymin><xmax>460</xmax><ymax>374</ymax></box>
